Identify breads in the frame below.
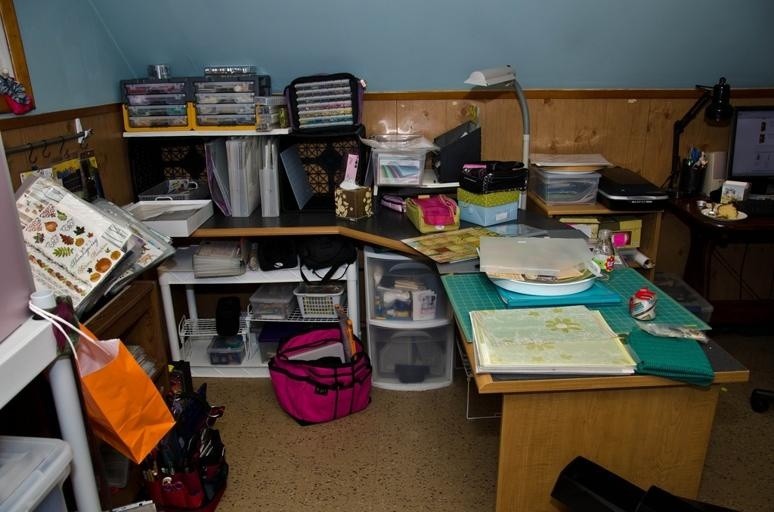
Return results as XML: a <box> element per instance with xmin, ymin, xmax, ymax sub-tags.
<box><xmin>717</xmin><ymin>203</ymin><xmax>738</xmax><ymax>219</ymax></box>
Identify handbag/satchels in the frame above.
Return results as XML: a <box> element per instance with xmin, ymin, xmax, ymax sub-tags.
<box><xmin>258</xmin><ymin>236</ymin><xmax>298</xmax><ymax>271</ymax></box>
<box><xmin>268</xmin><ymin>328</ymin><xmax>373</xmax><ymax>426</ymax></box>
<box><xmin>29</xmin><ymin>300</ymin><xmax>229</xmax><ymax>511</ymax></box>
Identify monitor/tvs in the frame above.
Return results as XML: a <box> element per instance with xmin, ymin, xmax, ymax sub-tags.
<box><xmin>726</xmin><ymin>106</ymin><xmax>774</xmax><ymax>200</ymax></box>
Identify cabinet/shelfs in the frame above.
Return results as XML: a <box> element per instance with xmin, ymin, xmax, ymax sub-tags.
<box><xmin>0</xmin><ymin>124</ymin><xmax>774</xmax><ymax>512</ymax></box>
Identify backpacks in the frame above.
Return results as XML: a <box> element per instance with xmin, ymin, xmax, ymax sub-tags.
<box><xmin>299</xmin><ymin>234</ymin><xmax>357</xmax><ymax>285</ymax></box>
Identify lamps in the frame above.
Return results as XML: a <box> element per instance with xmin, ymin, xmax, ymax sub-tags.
<box><xmin>659</xmin><ymin>75</ymin><xmax>740</xmax><ymax>202</ymax></box>
<box><xmin>464</xmin><ymin>62</ymin><xmax>531</xmax><ymax>213</ymax></box>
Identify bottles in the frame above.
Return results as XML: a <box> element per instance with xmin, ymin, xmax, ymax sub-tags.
<box><xmin>590</xmin><ymin>229</ymin><xmax>615</xmax><ymax>283</ymax></box>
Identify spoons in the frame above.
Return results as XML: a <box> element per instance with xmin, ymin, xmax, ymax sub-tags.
<box><xmin>708</xmin><ymin>202</ymin><xmax>716</xmax><ymax>217</ymax></box>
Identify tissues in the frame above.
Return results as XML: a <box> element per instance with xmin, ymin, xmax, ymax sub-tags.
<box><xmin>333</xmin><ymin>181</ymin><xmax>375</xmax><ymax>221</ymax></box>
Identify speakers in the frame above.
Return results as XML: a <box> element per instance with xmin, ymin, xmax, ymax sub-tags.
<box><xmin>700</xmin><ymin>151</ymin><xmax>728</xmax><ymax>198</ymax></box>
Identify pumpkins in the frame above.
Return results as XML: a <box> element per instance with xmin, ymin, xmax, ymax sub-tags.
<box><xmin>95</xmin><ymin>258</ymin><xmax>111</xmax><ymax>273</ymax></box>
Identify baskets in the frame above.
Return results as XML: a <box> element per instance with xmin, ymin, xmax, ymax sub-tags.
<box><xmin>293</xmin><ymin>282</ymin><xmax>347</xmax><ymax>318</ymax></box>
<box><xmin>137</xmin><ymin>179</ymin><xmax>209</xmax><ymax>201</ymax></box>
<box><xmin>405</xmin><ymin>197</ymin><xmax>460</xmax><ymax>233</ymax></box>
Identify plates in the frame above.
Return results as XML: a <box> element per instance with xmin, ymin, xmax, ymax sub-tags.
<box><xmin>701</xmin><ymin>208</ymin><xmax>748</xmax><ymax>222</ymax></box>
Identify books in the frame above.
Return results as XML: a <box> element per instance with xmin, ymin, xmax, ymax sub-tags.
<box><xmin>202</xmin><ymin>136</ymin><xmax>288</xmax><ymax>218</ymax></box>
<box><xmin>467</xmin><ymin>304</ymin><xmax>637</xmax><ymax>378</ymax></box>
<box><xmin>192</xmin><ymin>237</ymin><xmax>246</xmax><ymax>277</ymax></box>
<box><xmin>528</xmin><ymin>153</ymin><xmax>614</xmax><ymax>168</ymax></box>
